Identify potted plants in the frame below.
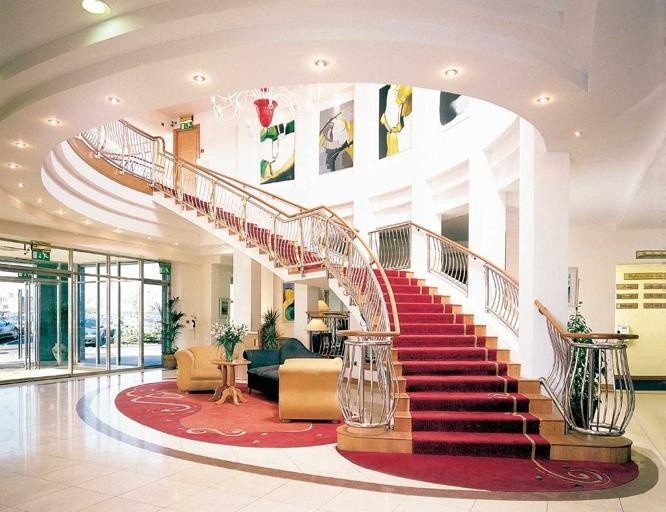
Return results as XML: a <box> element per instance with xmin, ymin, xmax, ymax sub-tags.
<box><xmin>46</xmin><ymin>299</ymin><xmax>70</xmax><ymax>365</ymax></box>
<box><xmin>140</xmin><ymin>295</ymin><xmax>185</xmax><ymax>370</ymax></box>
<box><xmin>566</xmin><ymin>299</ymin><xmax>604</xmax><ymax>429</ymax></box>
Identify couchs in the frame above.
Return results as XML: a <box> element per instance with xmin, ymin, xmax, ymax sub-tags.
<box><xmin>173</xmin><ymin>342</ymin><xmax>257</xmax><ymax>396</ymax></box>
<box><xmin>242</xmin><ymin>338</ymin><xmax>326</xmax><ymax>397</ymax></box>
<box><xmin>278</xmin><ymin>358</ymin><xmax>346</xmax><ymax>423</ymax></box>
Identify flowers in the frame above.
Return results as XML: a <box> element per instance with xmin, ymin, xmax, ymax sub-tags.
<box><xmin>211</xmin><ymin>315</ymin><xmax>248</xmax><ymax>350</ymax></box>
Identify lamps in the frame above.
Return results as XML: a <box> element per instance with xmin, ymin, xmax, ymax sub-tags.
<box><xmin>209</xmin><ymin>85</ymin><xmax>301</xmax><ymax>140</ymax></box>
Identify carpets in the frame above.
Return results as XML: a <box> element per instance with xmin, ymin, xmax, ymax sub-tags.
<box><xmin>114</xmin><ymin>381</ymin><xmax>344</xmax><ymax>446</ymax></box>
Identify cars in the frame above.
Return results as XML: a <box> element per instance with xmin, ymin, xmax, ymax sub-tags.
<box><xmin>85</xmin><ymin>319</ymin><xmax>107</xmax><ymax>347</ymax></box>
<box><xmin>0</xmin><ymin>319</ymin><xmax>19</xmax><ymax>341</ymax></box>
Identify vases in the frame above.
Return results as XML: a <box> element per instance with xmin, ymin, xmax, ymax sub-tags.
<box><xmin>224</xmin><ymin>342</ymin><xmax>236</xmax><ymax>364</ymax></box>
